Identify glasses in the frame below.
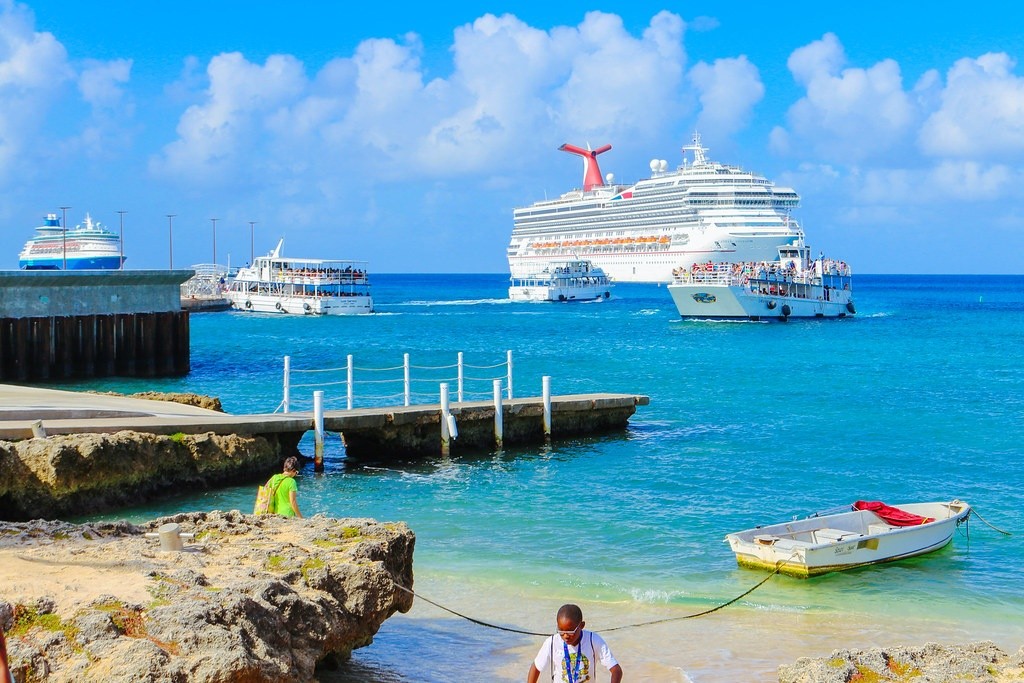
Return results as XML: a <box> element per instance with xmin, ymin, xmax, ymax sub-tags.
<box><xmin>555</xmin><ymin>622</ymin><xmax>581</xmax><ymax>636</ymax></box>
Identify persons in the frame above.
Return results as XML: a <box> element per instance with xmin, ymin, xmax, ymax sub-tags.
<box><xmin>266</xmin><ymin>456</ymin><xmax>303</xmax><ymax>518</ymax></box>
<box><xmin>273</xmin><ymin>267</ymin><xmax>369</xmax><ymax>298</ymax></box>
<box><xmin>671</xmin><ymin>250</ymin><xmax>850</xmax><ymax>302</ymax></box>
<box><xmin>527</xmin><ymin>604</ymin><xmax>623</xmax><ymax>683</ymax></box>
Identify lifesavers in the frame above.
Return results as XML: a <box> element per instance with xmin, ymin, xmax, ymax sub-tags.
<box><xmin>245</xmin><ymin>300</ymin><xmax>251</xmax><ymax>308</ymax></box>
<box><xmin>275</xmin><ymin>302</ymin><xmax>281</xmax><ymax>310</ymax></box>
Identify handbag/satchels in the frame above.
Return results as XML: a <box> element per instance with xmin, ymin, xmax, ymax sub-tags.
<box><xmin>253</xmin><ymin>485</ymin><xmax>274</xmax><ymax>515</ymax></box>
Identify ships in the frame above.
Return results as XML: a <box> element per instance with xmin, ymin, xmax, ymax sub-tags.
<box><xmin>505</xmin><ymin>128</ymin><xmax>808</xmax><ymax>284</ymax></box>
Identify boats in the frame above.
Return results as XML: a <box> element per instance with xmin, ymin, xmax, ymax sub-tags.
<box><xmin>667</xmin><ymin>240</ymin><xmax>856</xmax><ymax>321</ymax></box>
<box><xmin>507</xmin><ymin>258</ymin><xmax>616</xmax><ymax>302</ymax></box>
<box><xmin>222</xmin><ymin>236</ymin><xmax>374</xmax><ymax>315</ymax></box>
<box><xmin>726</xmin><ymin>499</ymin><xmax>971</xmax><ymax>579</ymax></box>
<box><xmin>17</xmin><ymin>213</ymin><xmax>127</xmax><ymax>271</ymax></box>
<box><xmin>179</xmin><ymin>263</ymin><xmax>241</xmax><ymax>312</ymax></box>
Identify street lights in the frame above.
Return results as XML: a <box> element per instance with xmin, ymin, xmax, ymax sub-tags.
<box><xmin>166</xmin><ymin>214</ymin><xmax>176</xmax><ymax>269</ymax></box>
<box><xmin>118</xmin><ymin>211</ymin><xmax>126</xmax><ymax>269</ymax></box>
<box><xmin>60</xmin><ymin>206</ymin><xmax>72</xmax><ymax>270</ymax></box>
<box><xmin>249</xmin><ymin>221</ymin><xmax>258</xmax><ymax>264</ymax></box>
<box><xmin>211</xmin><ymin>218</ymin><xmax>221</xmax><ymax>266</ymax></box>
